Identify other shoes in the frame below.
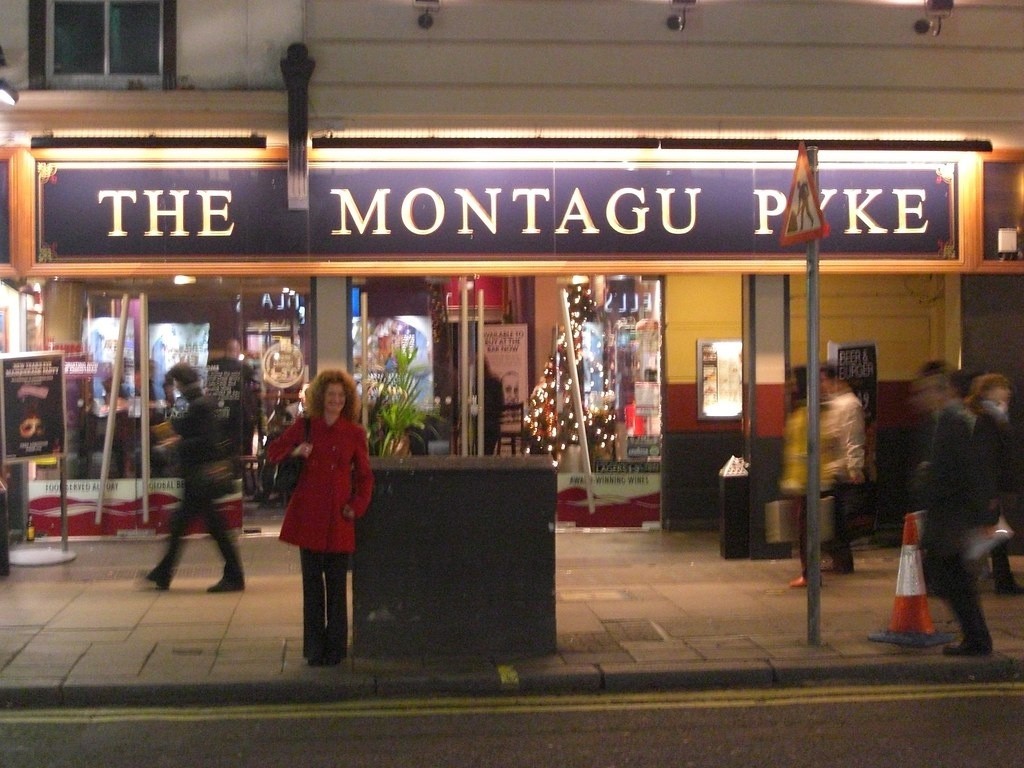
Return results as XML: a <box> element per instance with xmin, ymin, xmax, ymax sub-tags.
<box><xmin>144</xmin><ymin>572</ymin><xmax>170</xmax><ymax>590</ymax></box>
<box><xmin>788</xmin><ymin>577</ymin><xmax>821</xmax><ymax>586</ymax></box>
<box><xmin>943</xmin><ymin>637</ymin><xmax>992</xmax><ymax>655</ymax></box>
<box><xmin>993</xmin><ymin>575</ymin><xmax>1024</xmax><ymax>597</ymax></box>
<box><xmin>206</xmin><ymin>580</ymin><xmax>246</xmax><ymax>594</ymax></box>
<box><xmin>823</xmin><ymin>556</ymin><xmax>855</xmax><ymax>573</ymax></box>
<box><xmin>310</xmin><ymin>652</ymin><xmax>340</xmax><ymax>668</ymax></box>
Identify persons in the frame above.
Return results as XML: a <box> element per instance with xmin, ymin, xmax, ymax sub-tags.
<box><xmin>100</xmin><ymin>335</ymin><xmax>286</xmax><ymax>476</ymax></box>
<box><xmin>499</xmin><ymin>371</ymin><xmax>520</xmax><ymax>404</ymax></box>
<box><xmin>265</xmin><ymin>371</ymin><xmax>374</xmax><ymax>669</ymax></box>
<box><xmin>908</xmin><ymin>360</ymin><xmax>1024</xmax><ymax>658</ymax></box>
<box><xmin>475</xmin><ymin>354</ymin><xmax>503</xmax><ymax>456</ymax></box>
<box><xmin>782</xmin><ymin>359</ymin><xmax>868</xmax><ymax>589</ymax></box>
<box><xmin>144</xmin><ymin>364</ymin><xmax>247</xmax><ymax>591</ymax></box>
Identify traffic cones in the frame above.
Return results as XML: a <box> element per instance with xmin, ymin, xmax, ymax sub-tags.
<box><xmin>865</xmin><ymin>513</ymin><xmax>959</xmax><ymax>650</ymax></box>
